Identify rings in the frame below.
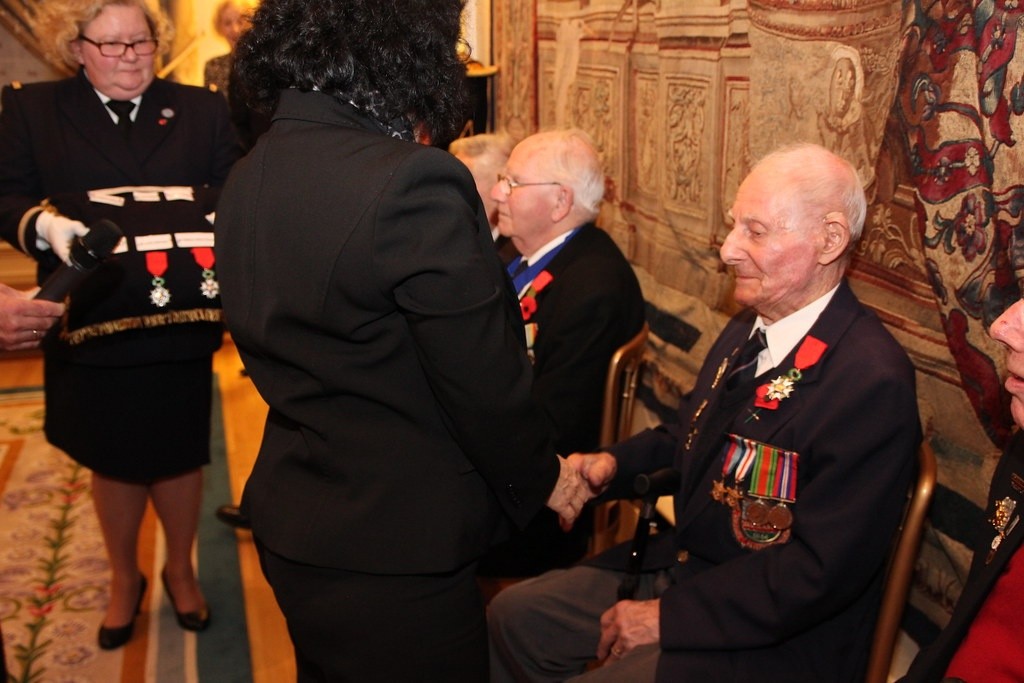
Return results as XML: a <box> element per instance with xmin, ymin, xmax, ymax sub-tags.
<box><xmin>33</xmin><ymin>330</ymin><xmax>38</xmax><ymax>339</ymax></box>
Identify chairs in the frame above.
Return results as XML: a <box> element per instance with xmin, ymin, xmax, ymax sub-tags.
<box><xmin>835</xmin><ymin>434</ymin><xmax>937</xmax><ymax>683</ymax></box>
<box><xmin>581</xmin><ymin>322</ymin><xmax>650</xmax><ymax>556</ymax></box>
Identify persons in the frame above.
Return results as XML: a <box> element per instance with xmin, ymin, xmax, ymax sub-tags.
<box><xmin>892</xmin><ymin>299</ymin><xmax>1024</xmax><ymax>683</ymax></box>
<box><xmin>450</xmin><ymin>129</ymin><xmax>645</xmax><ymax>588</ymax></box>
<box><xmin>487</xmin><ymin>142</ymin><xmax>924</xmax><ymax>683</ymax></box>
<box><xmin>0</xmin><ymin>0</ymin><xmax>251</xmax><ymax>651</ymax></box>
<box><xmin>215</xmin><ymin>0</ymin><xmax>593</xmax><ymax>683</ymax></box>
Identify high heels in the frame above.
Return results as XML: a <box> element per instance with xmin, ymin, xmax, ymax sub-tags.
<box><xmin>98</xmin><ymin>572</ymin><xmax>146</xmax><ymax>649</ymax></box>
<box><xmin>162</xmin><ymin>568</ymin><xmax>211</xmax><ymax>632</ymax></box>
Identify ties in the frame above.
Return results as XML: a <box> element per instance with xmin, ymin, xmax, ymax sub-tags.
<box><xmin>106</xmin><ymin>100</ymin><xmax>137</xmax><ymax>141</ymax></box>
<box><xmin>723</xmin><ymin>327</ymin><xmax>769</xmax><ymax>396</ymax></box>
<box><xmin>514</xmin><ymin>260</ymin><xmax>528</xmax><ymax>277</ymax></box>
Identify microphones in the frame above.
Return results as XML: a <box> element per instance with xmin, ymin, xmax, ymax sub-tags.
<box><xmin>31</xmin><ymin>219</ymin><xmax>124</xmax><ymax>304</ymax></box>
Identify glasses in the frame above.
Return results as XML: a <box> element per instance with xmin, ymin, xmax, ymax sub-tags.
<box><xmin>79</xmin><ymin>32</ymin><xmax>158</xmax><ymax>59</ymax></box>
<box><xmin>497</xmin><ymin>174</ymin><xmax>562</xmax><ymax>196</ymax></box>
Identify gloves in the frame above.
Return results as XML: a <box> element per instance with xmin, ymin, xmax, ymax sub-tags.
<box><xmin>36</xmin><ymin>211</ymin><xmax>89</xmax><ymax>269</ymax></box>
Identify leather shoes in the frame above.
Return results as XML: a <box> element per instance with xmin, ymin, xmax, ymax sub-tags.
<box><xmin>216</xmin><ymin>506</ymin><xmax>251</xmax><ymax>526</ymax></box>
